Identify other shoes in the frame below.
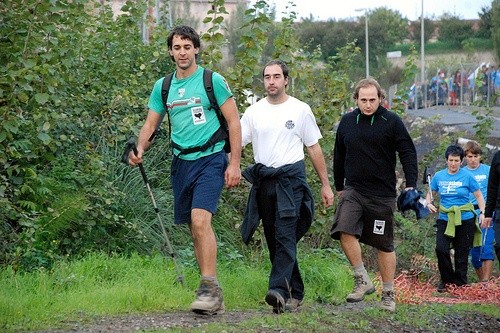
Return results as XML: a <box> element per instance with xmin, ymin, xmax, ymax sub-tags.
<box><xmin>437</xmin><ymin>280</ymin><xmax>449</xmax><ymax>292</ymax></box>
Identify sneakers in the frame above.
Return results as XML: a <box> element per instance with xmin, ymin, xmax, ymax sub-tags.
<box><xmin>285</xmin><ymin>297</ymin><xmax>304</xmax><ymax>313</ymax></box>
<box><xmin>379</xmin><ymin>291</ymin><xmax>395</xmax><ymax>313</ymax></box>
<box><xmin>346</xmin><ymin>271</ymin><xmax>376</xmax><ymax>303</ymax></box>
<box><xmin>190</xmin><ymin>280</ymin><xmax>225</xmax><ymax>316</ymax></box>
<box><xmin>265</xmin><ymin>290</ymin><xmax>285</xmax><ymax>314</ymax></box>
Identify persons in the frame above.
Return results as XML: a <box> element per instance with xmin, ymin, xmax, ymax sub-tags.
<box><xmin>329</xmin><ymin>79</ymin><xmax>418</xmax><ymax>315</ymax></box>
<box><xmin>227</xmin><ymin>59</ymin><xmax>334</xmax><ymax>315</ymax></box>
<box><xmin>483</xmin><ymin>149</ymin><xmax>500</xmax><ymax>269</ymax></box>
<box><xmin>384</xmin><ymin>64</ymin><xmax>500</xmax><ymax>110</ymax></box>
<box><xmin>462</xmin><ymin>140</ymin><xmax>495</xmax><ymax>283</ymax></box>
<box><xmin>128</xmin><ymin>25</ymin><xmax>242</xmax><ymax>315</ymax></box>
<box><xmin>426</xmin><ymin>145</ymin><xmax>486</xmax><ymax>293</ymax></box>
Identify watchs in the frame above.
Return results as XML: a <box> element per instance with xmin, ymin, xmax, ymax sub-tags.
<box><xmin>479</xmin><ymin>209</ymin><xmax>485</xmax><ymax>215</ymax></box>
